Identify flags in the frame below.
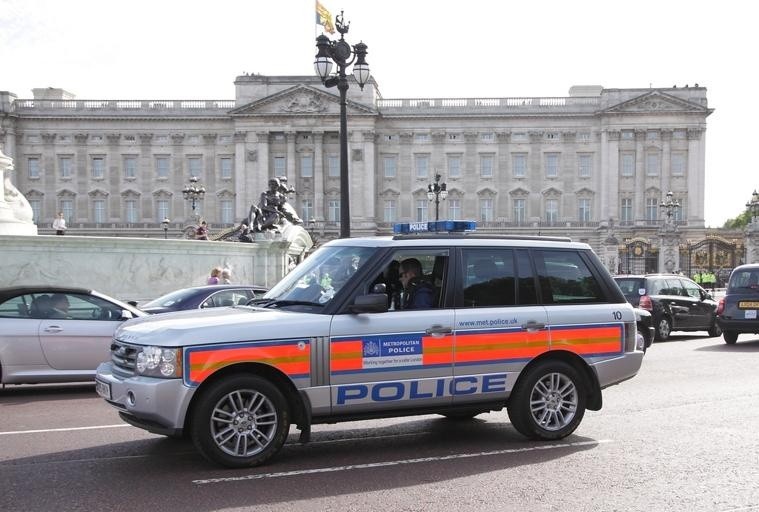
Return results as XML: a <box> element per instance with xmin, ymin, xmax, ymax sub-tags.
<box><xmin>316</xmin><ymin>2</ymin><xmax>335</xmax><ymax>34</ymax></box>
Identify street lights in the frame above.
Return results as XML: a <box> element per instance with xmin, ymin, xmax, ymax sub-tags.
<box><xmin>312</xmin><ymin>13</ymin><xmax>369</xmax><ymax>238</ymax></box>
<box><xmin>161</xmin><ymin>216</ymin><xmax>171</xmax><ymax>237</ymax></box>
<box><xmin>181</xmin><ymin>177</ymin><xmax>205</xmax><ymax>222</ymax></box>
<box><xmin>428</xmin><ymin>173</ymin><xmax>449</xmax><ymax>219</ymax></box>
<box><xmin>745</xmin><ymin>189</ymin><xmax>759</xmax><ymax>219</ymax></box>
<box><xmin>659</xmin><ymin>191</ymin><xmax>681</xmax><ymax>231</ymax></box>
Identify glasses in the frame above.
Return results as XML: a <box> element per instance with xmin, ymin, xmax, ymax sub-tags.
<box><xmin>399</xmin><ymin>270</ymin><xmax>412</xmax><ymax>278</ymax></box>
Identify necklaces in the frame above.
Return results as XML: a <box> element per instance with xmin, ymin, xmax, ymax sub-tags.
<box><xmin>198</xmin><ymin>222</ymin><xmax>209</xmax><ymax>240</ymax></box>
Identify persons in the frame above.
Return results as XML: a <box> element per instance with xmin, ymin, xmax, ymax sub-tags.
<box><xmin>53</xmin><ymin>212</ymin><xmax>67</xmax><ymax>235</ymax></box>
<box><xmin>208</xmin><ymin>268</ymin><xmax>221</xmax><ymax>285</ymax></box>
<box><xmin>221</xmin><ymin>270</ymin><xmax>231</xmax><ymax>284</ymax></box>
<box><xmin>332</xmin><ymin>255</ymin><xmax>360</xmax><ymax>292</ymax></box>
<box><xmin>239</xmin><ymin>178</ymin><xmax>287</xmax><ymax>243</ymax></box>
<box><xmin>398</xmin><ymin>258</ymin><xmax>435</xmax><ymax>309</ymax></box>
<box><xmin>48</xmin><ymin>294</ymin><xmax>72</xmax><ymax>319</ymax></box>
<box><xmin>30</xmin><ymin>295</ymin><xmax>50</xmax><ymax>317</ymax></box>
